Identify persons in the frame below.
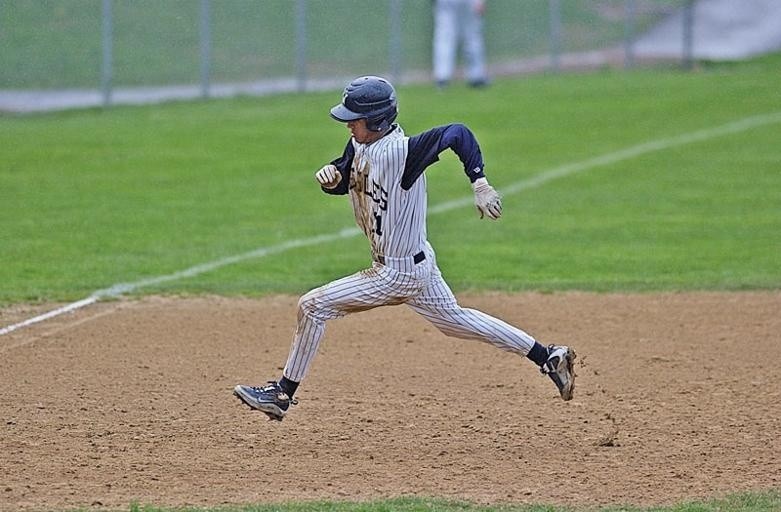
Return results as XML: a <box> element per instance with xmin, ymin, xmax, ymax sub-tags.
<box><xmin>233</xmin><ymin>75</ymin><xmax>577</xmax><ymax>423</ymax></box>
<box><xmin>433</xmin><ymin>0</ymin><xmax>487</xmax><ymax>88</ymax></box>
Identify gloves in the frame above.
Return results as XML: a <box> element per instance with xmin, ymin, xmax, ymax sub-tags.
<box><xmin>314</xmin><ymin>163</ymin><xmax>343</xmax><ymax>189</ymax></box>
<box><xmin>470</xmin><ymin>176</ymin><xmax>504</xmax><ymax>221</ymax></box>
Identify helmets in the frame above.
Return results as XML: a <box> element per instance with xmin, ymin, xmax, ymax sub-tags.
<box><xmin>328</xmin><ymin>74</ymin><xmax>399</xmax><ymax>133</ymax></box>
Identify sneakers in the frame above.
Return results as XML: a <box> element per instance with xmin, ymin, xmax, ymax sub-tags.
<box><xmin>539</xmin><ymin>342</ymin><xmax>577</xmax><ymax>403</ymax></box>
<box><xmin>234</xmin><ymin>381</ymin><xmax>299</xmax><ymax>422</ymax></box>
